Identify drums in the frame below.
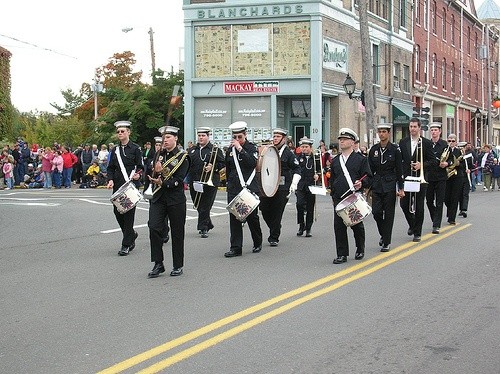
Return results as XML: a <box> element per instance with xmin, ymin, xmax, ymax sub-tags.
<box><xmin>256</xmin><ymin>145</ymin><xmax>281</xmax><ymax>198</ymax></box>
<box><xmin>110</xmin><ymin>180</ymin><xmax>143</xmax><ymax>214</ymax></box>
<box><xmin>335</xmin><ymin>192</ymin><xmax>372</xmax><ymax>227</ymax></box>
<box><xmin>226</xmin><ymin>188</ymin><xmax>260</xmax><ymax>221</ymax></box>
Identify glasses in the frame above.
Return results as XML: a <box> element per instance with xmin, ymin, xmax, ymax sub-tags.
<box><xmin>448</xmin><ymin>140</ymin><xmax>455</xmax><ymax>142</ymax></box>
<box><xmin>116</xmin><ymin>130</ymin><xmax>124</xmax><ymax>133</ymax></box>
<box><xmin>232</xmin><ymin>135</ymin><xmax>243</xmax><ymax>139</ymax></box>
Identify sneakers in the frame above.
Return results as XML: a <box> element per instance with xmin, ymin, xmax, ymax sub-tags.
<box><xmin>484</xmin><ymin>188</ymin><xmax>488</xmax><ymax>192</ymax></box>
<box><xmin>476</xmin><ymin>183</ymin><xmax>482</xmax><ymax>185</ymax></box>
<box><xmin>484</xmin><ymin>186</ymin><xmax>486</xmax><ymax>189</ymax></box>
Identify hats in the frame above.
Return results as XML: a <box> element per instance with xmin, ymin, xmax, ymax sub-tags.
<box><xmin>229</xmin><ymin>120</ymin><xmax>247</xmax><ymax>132</ymax></box>
<box><xmin>377</xmin><ymin>123</ymin><xmax>393</xmax><ymax>128</ymax></box>
<box><xmin>274</xmin><ymin>128</ymin><xmax>288</xmax><ymax>135</ymax></box>
<box><xmin>337</xmin><ymin>128</ymin><xmax>358</xmax><ymax>141</ymax></box>
<box><xmin>300</xmin><ymin>139</ymin><xmax>315</xmax><ymax>144</ymax></box>
<box><xmin>196</xmin><ymin>127</ymin><xmax>211</xmax><ymax>132</ymax></box>
<box><xmin>114</xmin><ymin>121</ymin><xmax>132</xmax><ymax>127</ymax></box>
<box><xmin>429</xmin><ymin>122</ymin><xmax>442</xmax><ymax>128</ymax></box>
<box><xmin>158</xmin><ymin>125</ymin><xmax>180</xmax><ymax>133</ymax></box>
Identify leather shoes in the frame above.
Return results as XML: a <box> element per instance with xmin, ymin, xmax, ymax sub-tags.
<box><xmin>381</xmin><ymin>244</ymin><xmax>390</xmax><ymax>251</ymax></box>
<box><xmin>432</xmin><ymin>226</ymin><xmax>439</xmax><ymax>234</ymax></box>
<box><xmin>297</xmin><ymin>223</ymin><xmax>305</xmax><ymax>235</ymax></box>
<box><xmin>129</xmin><ymin>232</ymin><xmax>138</xmax><ymax>251</ymax></box>
<box><xmin>170</xmin><ymin>267</ymin><xmax>182</xmax><ymax>276</ymax></box>
<box><xmin>459</xmin><ymin>211</ymin><xmax>467</xmax><ymax>218</ymax></box>
<box><xmin>253</xmin><ymin>244</ymin><xmax>262</xmax><ymax>253</ymax></box>
<box><xmin>408</xmin><ymin>229</ymin><xmax>414</xmax><ymax>235</ymax></box>
<box><xmin>225</xmin><ymin>248</ymin><xmax>242</xmax><ymax>257</ymax></box>
<box><xmin>450</xmin><ymin>220</ymin><xmax>456</xmax><ymax>224</ymax></box>
<box><xmin>119</xmin><ymin>245</ymin><xmax>129</xmax><ymax>256</ymax></box>
<box><xmin>379</xmin><ymin>238</ymin><xmax>383</xmax><ymax>246</ymax></box>
<box><xmin>201</xmin><ymin>230</ymin><xmax>208</xmax><ymax>238</ymax></box>
<box><xmin>199</xmin><ymin>224</ymin><xmax>214</xmax><ymax>234</ymax></box>
<box><xmin>163</xmin><ymin>227</ymin><xmax>169</xmax><ymax>243</ymax></box>
<box><xmin>413</xmin><ymin>236</ymin><xmax>421</xmax><ymax>242</ymax></box>
<box><xmin>333</xmin><ymin>255</ymin><xmax>347</xmax><ymax>264</ymax></box>
<box><xmin>306</xmin><ymin>230</ymin><xmax>312</xmax><ymax>237</ymax></box>
<box><xmin>355</xmin><ymin>248</ymin><xmax>364</xmax><ymax>259</ymax></box>
<box><xmin>270</xmin><ymin>242</ymin><xmax>278</xmax><ymax>247</ymax></box>
<box><xmin>148</xmin><ymin>262</ymin><xmax>165</xmax><ymax>277</ymax></box>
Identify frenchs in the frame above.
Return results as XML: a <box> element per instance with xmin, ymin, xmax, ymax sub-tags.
<box><xmin>440</xmin><ymin>145</ymin><xmax>450</xmax><ymax>162</ymax></box>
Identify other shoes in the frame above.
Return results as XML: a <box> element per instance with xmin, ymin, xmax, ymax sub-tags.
<box><xmin>492</xmin><ymin>188</ymin><xmax>494</xmax><ymax>191</ymax></box>
<box><xmin>498</xmin><ymin>188</ymin><xmax>500</xmax><ymax>191</ymax></box>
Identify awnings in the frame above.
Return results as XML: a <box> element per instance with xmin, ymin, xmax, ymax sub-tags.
<box><xmin>393</xmin><ymin>104</ymin><xmax>427</xmax><ymax>121</ymax></box>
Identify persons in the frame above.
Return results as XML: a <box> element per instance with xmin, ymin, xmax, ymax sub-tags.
<box><xmin>138</xmin><ymin>136</ymin><xmax>166</xmax><ymax>189</ymax></box>
<box><xmin>457</xmin><ymin>142</ymin><xmax>472</xmax><ymax>218</ymax></box>
<box><xmin>107</xmin><ymin>121</ymin><xmax>145</xmax><ymax>256</ymax></box>
<box><xmin>224</xmin><ymin>121</ymin><xmax>262</xmax><ymax>257</ymax></box>
<box><xmin>399</xmin><ymin>118</ymin><xmax>436</xmax><ymax>242</ymax></box>
<box><xmin>189</xmin><ymin>127</ymin><xmax>226</xmax><ymax>238</ymax></box>
<box><xmin>259</xmin><ymin>130</ymin><xmax>302</xmax><ymax>246</ymax></box>
<box><xmin>330</xmin><ymin>128</ymin><xmax>373</xmax><ymax>264</ymax></box>
<box><xmin>369</xmin><ymin>123</ymin><xmax>406</xmax><ymax>252</ymax></box>
<box><xmin>295</xmin><ymin>139</ymin><xmax>315</xmax><ymax>221</ymax></box>
<box><xmin>426</xmin><ymin>122</ymin><xmax>454</xmax><ymax>234</ymax></box>
<box><xmin>147</xmin><ymin>126</ymin><xmax>191</xmax><ymax>277</ymax></box>
<box><xmin>445</xmin><ymin>134</ymin><xmax>464</xmax><ymax>225</ymax></box>
<box><xmin>234</xmin><ymin>135</ymin><xmax>380</xmax><ymax>227</ymax></box>
<box><xmin>460</xmin><ymin>138</ymin><xmax>500</xmax><ymax>192</ymax></box>
<box><xmin>297</xmin><ymin>140</ymin><xmax>321</xmax><ymax>237</ymax></box>
<box><xmin>0</xmin><ymin>136</ymin><xmax>115</xmax><ymax>190</ymax></box>
<box><xmin>143</xmin><ymin>137</ymin><xmax>169</xmax><ymax>243</ymax></box>
<box><xmin>176</xmin><ymin>140</ymin><xmax>231</xmax><ymax>190</ymax></box>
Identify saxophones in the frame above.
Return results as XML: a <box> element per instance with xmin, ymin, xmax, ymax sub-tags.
<box><xmin>446</xmin><ymin>150</ymin><xmax>464</xmax><ymax>178</ymax></box>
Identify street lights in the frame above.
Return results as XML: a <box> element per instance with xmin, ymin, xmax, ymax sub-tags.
<box><xmin>470</xmin><ymin>107</ymin><xmax>487</xmax><ymax>119</ymax></box>
<box><xmin>343</xmin><ymin>74</ymin><xmax>364</xmax><ymax>106</ymax></box>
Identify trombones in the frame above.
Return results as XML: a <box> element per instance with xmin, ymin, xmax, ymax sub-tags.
<box><xmin>313</xmin><ymin>149</ymin><xmax>326</xmax><ymax>223</ymax></box>
<box><xmin>193</xmin><ymin>142</ymin><xmax>219</xmax><ymax>210</ymax></box>
<box><xmin>409</xmin><ymin>137</ymin><xmax>428</xmax><ymax>213</ymax></box>
